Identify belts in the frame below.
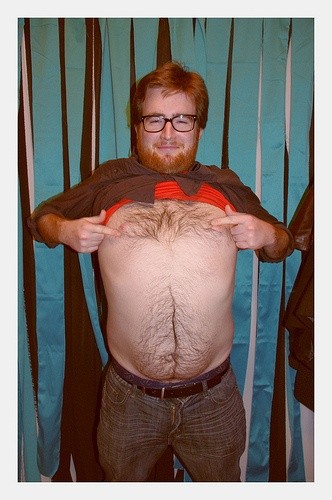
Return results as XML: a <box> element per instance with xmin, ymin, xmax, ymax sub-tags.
<box><xmin>110</xmin><ymin>358</ymin><xmax>231</xmax><ymax>399</ymax></box>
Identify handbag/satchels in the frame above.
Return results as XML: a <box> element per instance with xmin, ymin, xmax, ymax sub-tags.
<box><xmin>287</xmin><ymin>182</ymin><xmax>314</xmax><ymax>252</ymax></box>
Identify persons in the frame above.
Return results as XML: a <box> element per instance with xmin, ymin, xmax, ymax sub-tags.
<box><xmin>30</xmin><ymin>61</ymin><xmax>295</xmax><ymax>481</ymax></box>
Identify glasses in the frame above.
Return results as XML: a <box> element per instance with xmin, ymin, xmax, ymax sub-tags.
<box><xmin>139</xmin><ymin>114</ymin><xmax>198</xmax><ymax>133</ymax></box>
